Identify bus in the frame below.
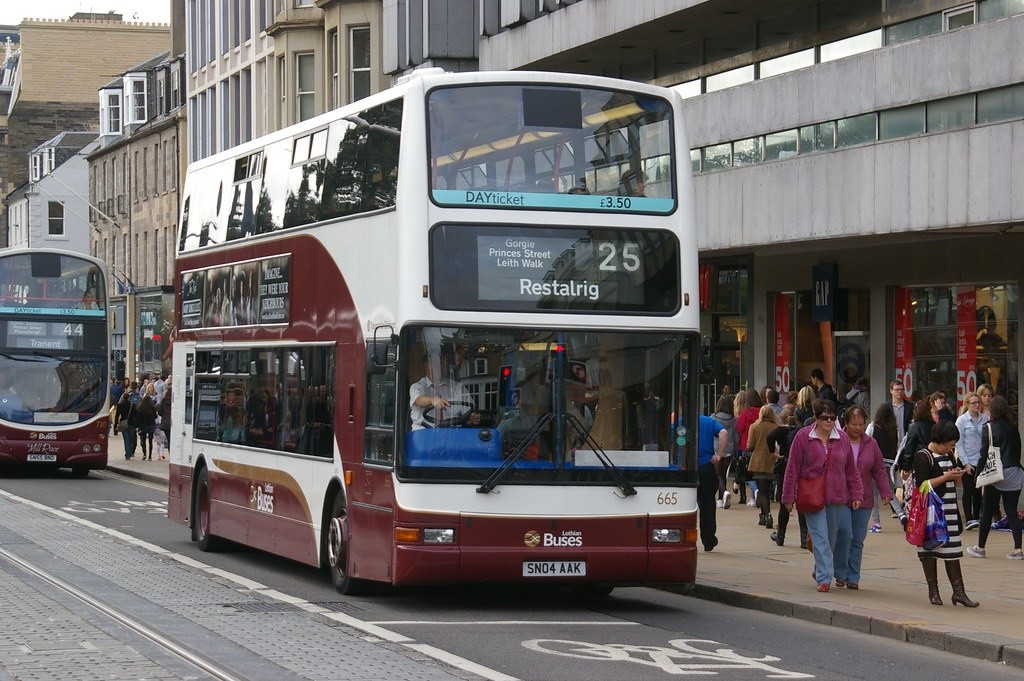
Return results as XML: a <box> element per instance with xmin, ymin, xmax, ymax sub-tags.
<box><xmin>166</xmin><ymin>62</ymin><xmax>705</xmax><ymax>604</ymax></box>
<box><xmin>0</xmin><ymin>248</ymin><xmax>111</xmax><ymax>476</ymax></box>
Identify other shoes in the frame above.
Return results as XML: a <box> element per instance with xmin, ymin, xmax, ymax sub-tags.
<box><xmin>705</xmin><ymin>535</ymin><xmax>718</xmax><ymax>551</ymax></box>
<box><xmin>898</xmin><ymin>512</ymin><xmax>907</xmax><ymax>532</ymax></box>
<box><xmin>965</xmin><ymin>519</ymin><xmax>981</xmax><ymax>530</ymax></box>
<box><xmin>800</xmin><ymin>538</ymin><xmax>807</xmax><ymax>548</ymax></box>
<box><xmin>747</xmin><ymin>489</ymin><xmax>761</xmax><ymax>508</ymax></box>
<box><xmin>771</xmin><ymin>531</ymin><xmax>784</xmax><ymax>546</ymax></box>
<box><xmin>718</xmin><ymin>491</ymin><xmax>732</xmax><ymax>509</ymax></box>
<box><xmin>733</xmin><ymin>480</ymin><xmax>738</xmax><ymax>494</ymax></box>
<box><xmin>1006</xmin><ymin>551</ymin><xmax>1024</xmax><ymax>560</ymax></box>
<box><xmin>758</xmin><ymin>513</ymin><xmax>773</xmax><ymax>529</ymax></box>
<box><xmin>967</xmin><ymin>545</ymin><xmax>985</xmax><ymax>558</ymax></box>
<box><xmin>817</xmin><ymin>583</ymin><xmax>829</xmax><ymax>592</ymax></box>
<box><xmin>835</xmin><ymin>580</ymin><xmax>860</xmax><ymax>589</ymax></box>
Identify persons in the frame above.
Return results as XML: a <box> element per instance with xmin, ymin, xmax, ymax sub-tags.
<box><xmin>111</xmin><ymin>372</ymin><xmax>172</xmax><ymax>463</ymax></box>
<box><xmin>568</xmin><ymin>370</ymin><xmax>1024</xmax><ymax>608</ymax></box>
<box><xmin>186</xmin><ymin>271</ymin><xmax>258</xmax><ymax>327</ymax></box>
<box><xmin>409</xmin><ymin>347</ymin><xmax>480</xmax><ymax>430</ymax></box>
<box><xmin>219</xmin><ymin>378</ymin><xmax>336</xmax><ymax>457</ymax></box>
<box><xmin>0</xmin><ymin>284</ymin><xmax>100</xmax><ymax>311</ymax></box>
<box><xmin>511</xmin><ymin>171</ymin><xmax>651</xmax><ymax>197</ymax></box>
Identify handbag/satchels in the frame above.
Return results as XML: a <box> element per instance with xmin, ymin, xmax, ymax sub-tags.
<box><xmin>733</xmin><ymin>449</ymin><xmax>757</xmax><ymax>481</ymax></box>
<box><xmin>975</xmin><ymin>423</ymin><xmax>1004</xmax><ymax>489</ymax></box>
<box><xmin>796</xmin><ymin>474</ymin><xmax>825</xmax><ymax>513</ymax></box>
<box><xmin>906</xmin><ymin>479</ymin><xmax>949</xmax><ymax>550</ymax></box>
<box><xmin>117</xmin><ymin>419</ymin><xmax>129</xmax><ymax>432</ymax></box>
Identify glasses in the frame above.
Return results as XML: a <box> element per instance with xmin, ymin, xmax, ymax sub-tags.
<box><xmin>819</xmin><ymin>415</ymin><xmax>837</xmax><ymax>421</ymax></box>
<box><xmin>892</xmin><ymin>389</ymin><xmax>905</xmax><ymax>392</ymax></box>
<box><xmin>968</xmin><ymin>400</ymin><xmax>982</xmax><ymax>405</ymax></box>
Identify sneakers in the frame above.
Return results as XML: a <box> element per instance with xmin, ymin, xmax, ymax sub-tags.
<box><xmin>867</xmin><ymin>524</ymin><xmax>882</xmax><ymax>533</ymax></box>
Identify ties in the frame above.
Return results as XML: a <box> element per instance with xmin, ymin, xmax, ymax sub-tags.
<box><xmin>435</xmin><ymin>388</ymin><xmax>441</xmax><ymax>397</ymax></box>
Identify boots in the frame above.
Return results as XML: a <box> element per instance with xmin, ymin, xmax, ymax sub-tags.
<box><xmin>945</xmin><ymin>559</ymin><xmax>980</xmax><ymax>608</ymax></box>
<box><xmin>920</xmin><ymin>558</ymin><xmax>943</xmax><ymax>605</ymax></box>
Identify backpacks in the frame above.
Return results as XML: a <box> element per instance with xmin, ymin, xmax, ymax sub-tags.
<box><xmin>904</xmin><ymin>449</ymin><xmax>954</xmax><ymax>519</ymax></box>
<box><xmin>837</xmin><ymin>390</ymin><xmax>864</xmax><ymax>426</ymax></box>
